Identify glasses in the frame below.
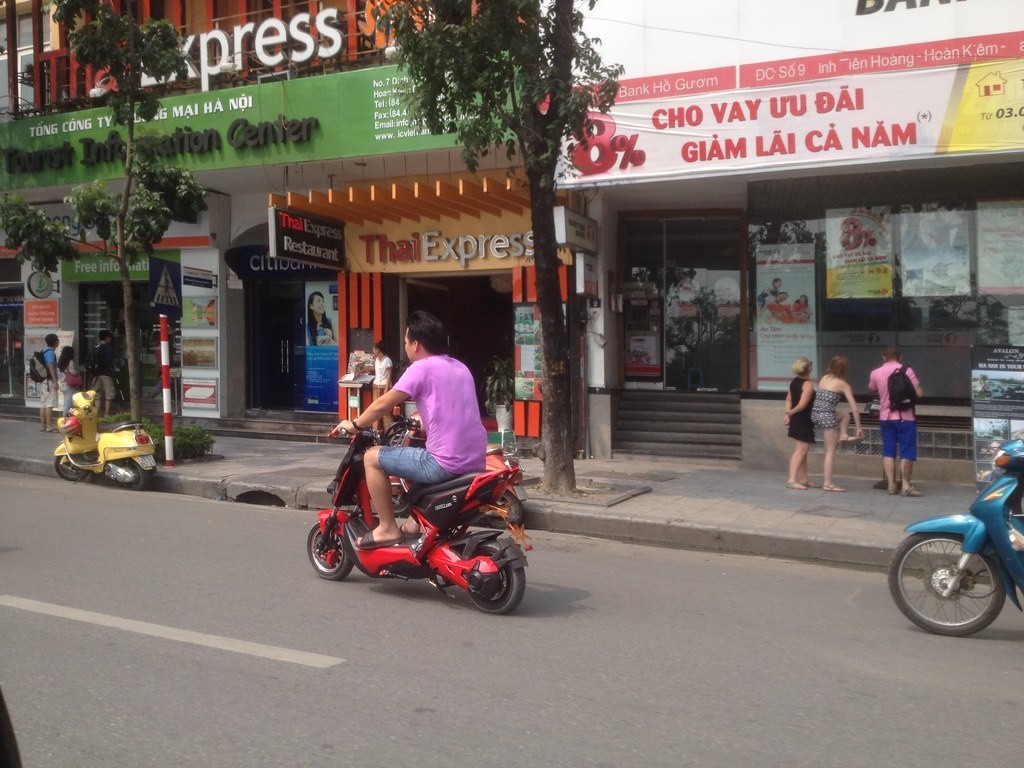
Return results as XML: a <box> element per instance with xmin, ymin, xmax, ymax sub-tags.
<box><xmin>810</xmin><ymin>361</ymin><xmax>813</xmax><ymax>366</ymax></box>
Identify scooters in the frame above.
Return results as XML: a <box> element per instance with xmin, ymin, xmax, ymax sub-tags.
<box><xmin>889</xmin><ymin>439</ymin><xmax>1024</xmax><ymax>637</ymax></box>
<box><xmin>53</xmin><ymin>388</ymin><xmax>158</xmax><ymax>492</ymax></box>
<box><xmin>388</xmin><ymin>410</ymin><xmax>529</xmax><ymax>529</ymax></box>
<box><xmin>306</xmin><ymin>413</ymin><xmax>534</xmax><ymax>615</ymax></box>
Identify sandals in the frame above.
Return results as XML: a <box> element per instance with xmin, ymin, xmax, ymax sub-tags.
<box><xmin>824</xmin><ymin>484</ymin><xmax>846</xmax><ymax>492</ymax></box>
<box><xmin>839</xmin><ymin>436</ymin><xmax>862</xmax><ymax>443</ymax></box>
<box><xmin>799</xmin><ymin>480</ymin><xmax>816</xmax><ymax>487</ymax></box>
<box><xmin>40</xmin><ymin>427</ymin><xmax>60</xmax><ymax>433</ymax></box>
<box><xmin>786</xmin><ymin>481</ymin><xmax>808</xmax><ymax>490</ymax></box>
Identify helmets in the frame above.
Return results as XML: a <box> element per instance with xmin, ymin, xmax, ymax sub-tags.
<box><xmin>64</xmin><ymin>417</ymin><xmax>81</xmax><ymax>434</ymax></box>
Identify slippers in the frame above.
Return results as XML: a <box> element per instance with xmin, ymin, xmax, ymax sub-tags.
<box><xmin>399</xmin><ymin>524</ymin><xmax>423</xmax><ymax>538</ymax></box>
<box><xmin>355</xmin><ymin>530</ymin><xmax>404</xmax><ymax>549</ymax></box>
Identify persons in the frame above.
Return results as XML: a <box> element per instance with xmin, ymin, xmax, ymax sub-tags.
<box><xmin>306</xmin><ymin>292</ymin><xmax>333</xmax><ymax>346</ymax></box>
<box><xmin>331</xmin><ymin>311</ymin><xmax>488</xmax><ymax>548</ymax></box>
<box><xmin>39</xmin><ymin>330</ymin><xmax>117</xmax><ymax>433</ymax></box>
<box><xmin>810</xmin><ymin>355</ymin><xmax>865</xmax><ymax>491</ymax></box>
<box><xmin>758</xmin><ymin>278</ymin><xmax>809</xmax><ymax>313</ymax></box>
<box><xmin>784</xmin><ymin>357</ymin><xmax>820</xmax><ymax>490</ymax></box>
<box><xmin>372</xmin><ymin>341</ymin><xmax>394</xmax><ymax>435</ymax></box>
<box><xmin>867</xmin><ymin>346</ymin><xmax>924</xmax><ymax>497</ymax></box>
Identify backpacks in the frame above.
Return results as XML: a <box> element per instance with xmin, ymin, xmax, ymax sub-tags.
<box><xmin>85</xmin><ymin>344</ymin><xmax>108</xmax><ymax>375</ymax></box>
<box><xmin>888</xmin><ymin>365</ymin><xmax>916</xmax><ymax>411</ymax></box>
<box><xmin>30</xmin><ymin>349</ymin><xmax>56</xmax><ymax>382</ymax></box>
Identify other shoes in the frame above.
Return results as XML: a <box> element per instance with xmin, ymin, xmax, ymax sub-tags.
<box><xmin>900</xmin><ymin>486</ymin><xmax>923</xmax><ymax>496</ymax></box>
<box><xmin>887</xmin><ymin>487</ymin><xmax>899</xmax><ymax>494</ymax></box>
<box><xmin>104</xmin><ymin>414</ymin><xmax>116</xmax><ymax>419</ymax></box>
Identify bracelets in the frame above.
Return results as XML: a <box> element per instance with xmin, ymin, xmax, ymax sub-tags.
<box><xmin>352</xmin><ymin>416</ymin><xmax>360</xmax><ymax>431</ymax></box>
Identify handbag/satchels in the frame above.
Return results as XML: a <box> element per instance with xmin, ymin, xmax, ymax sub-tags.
<box><xmin>66</xmin><ymin>366</ymin><xmax>82</xmax><ymax>387</ymax></box>
